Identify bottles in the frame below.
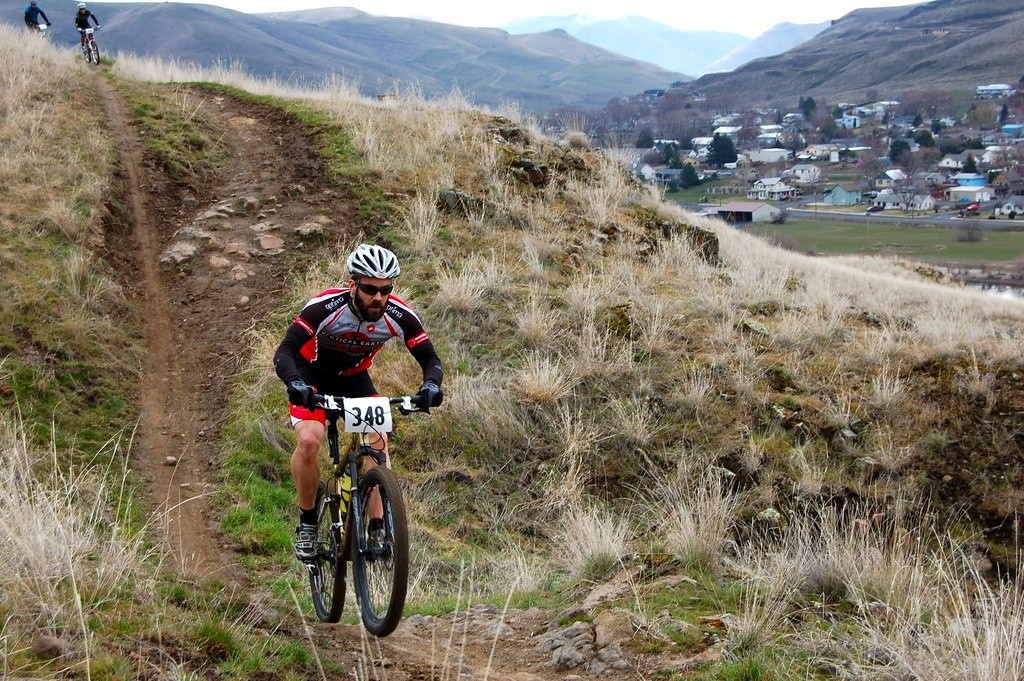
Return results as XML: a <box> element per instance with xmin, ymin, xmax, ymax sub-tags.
<box><xmin>340</xmin><ymin>465</ymin><xmax>353</xmax><ymax>516</ymax></box>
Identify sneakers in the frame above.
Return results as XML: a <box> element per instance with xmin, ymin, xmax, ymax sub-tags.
<box><xmin>294</xmin><ymin>524</ymin><xmax>320</xmax><ymax>563</ymax></box>
<box><xmin>367</xmin><ymin>529</ymin><xmax>387</xmax><ymax>549</ymax></box>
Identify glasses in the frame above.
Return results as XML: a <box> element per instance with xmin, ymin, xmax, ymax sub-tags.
<box><xmin>80</xmin><ymin>8</ymin><xmax>85</xmax><ymax>10</ymax></box>
<box><xmin>352</xmin><ymin>280</ymin><xmax>394</xmax><ymax>296</ymax></box>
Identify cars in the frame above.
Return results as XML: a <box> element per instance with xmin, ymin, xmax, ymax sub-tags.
<box><xmin>966</xmin><ymin>202</ymin><xmax>979</xmax><ymax>212</ymax></box>
<box><xmin>867</xmin><ymin>205</ymin><xmax>882</xmax><ymax>212</ymax></box>
<box><xmin>779</xmin><ymin>194</ymin><xmax>788</xmax><ymax>201</ymax></box>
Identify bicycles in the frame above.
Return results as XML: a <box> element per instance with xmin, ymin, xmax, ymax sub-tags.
<box><xmin>77</xmin><ymin>25</ymin><xmax>101</xmax><ymax>66</ymax></box>
<box><xmin>32</xmin><ymin>22</ymin><xmax>53</xmax><ymax>44</ymax></box>
<box><xmin>293</xmin><ymin>381</ymin><xmax>435</xmax><ymax>638</ymax></box>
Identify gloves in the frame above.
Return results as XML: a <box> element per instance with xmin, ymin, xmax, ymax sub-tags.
<box><xmin>415</xmin><ymin>381</ymin><xmax>444</xmax><ymax>415</ymax></box>
<box><xmin>285</xmin><ymin>372</ymin><xmax>316</xmax><ymax>412</ymax></box>
<box><xmin>47</xmin><ymin>22</ymin><xmax>51</xmax><ymax>26</ymax></box>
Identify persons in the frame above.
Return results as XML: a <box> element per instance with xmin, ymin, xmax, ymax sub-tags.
<box><xmin>75</xmin><ymin>2</ymin><xmax>100</xmax><ymax>52</ymax></box>
<box><xmin>24</xmin><ymin>1</ymin><xmax>51</xmax><ymax>35</ymax></box>
<box><xmin>272</xmin><ymin>245</ymin><xmax>445</xmax><ymax>561</ymax></box>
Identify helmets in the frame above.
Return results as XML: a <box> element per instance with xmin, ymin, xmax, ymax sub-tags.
<box><xmin>30</xmin><ymin>0</ymin><xmax>37</xmax><ymax>5</ymax></box>
<box><xmin>347</xmin><ymin>243</ymin><xmax>401</xmax><ymax>280</ymax></box>
<box><xmin>77</xmin><ymin>2</ymin><xmax>87</xmax><ymax>8</ymax></box>
